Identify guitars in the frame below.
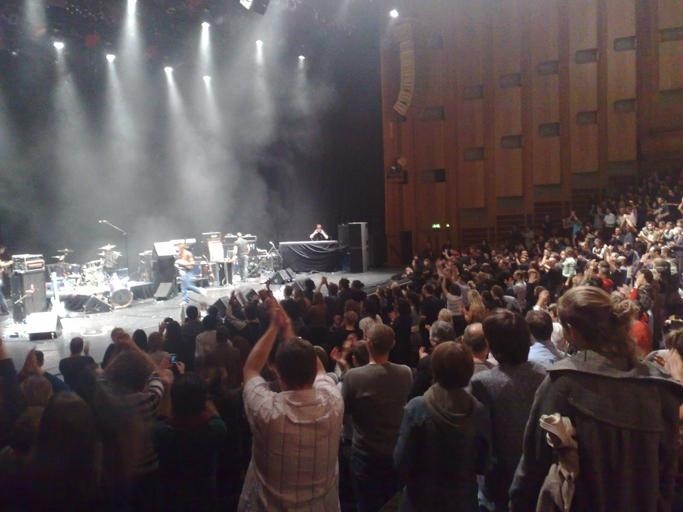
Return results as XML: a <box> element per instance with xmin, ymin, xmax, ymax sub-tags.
<box><xmin>49</xmin><ymin>272</ymin><xmax>68</xmax><ymax>317</ymax></box>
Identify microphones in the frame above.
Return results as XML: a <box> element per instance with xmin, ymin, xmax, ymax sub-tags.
<box><xmin>59</xmin><ymin>248</ymin><xmax>74</xmax><ymax>255</ymax></box>
<box><xmin>98</xmin><ymin>219</ymin><xmax>107</xmax><ymax>225</ymax></box>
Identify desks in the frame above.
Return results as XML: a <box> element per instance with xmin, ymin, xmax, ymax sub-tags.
<box><xmin>278</xmin><ymin>240</ymin><xmax>341</xmax><ymax>272</ymax></box>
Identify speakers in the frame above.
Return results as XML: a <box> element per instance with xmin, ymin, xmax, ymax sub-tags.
<box><xmin>272</xmin><ymin>267</ymin><xmax>313</xmax><ymax>298</ymax></box>
<box><xmin>347</xmin><ymin>222</ymin><xmax>369</xmax><ymax>273</ymax></box>
<box><xmin>26</xmin><ymin>312</ymin><xmax>63</xmax><ymax>341</ymax></box>
<box><xmin>435</xmin><ymin>168</ymin><xmax>445</xmax><ymax>182</ymax></box>
<box><xmin>11</xmin><ymin>254</ymin><xmax>45</xmax><ymax>323</ymax></box>
<box><xmin>268</xmin><ymin>241</ymin><xmax>274</xmax><ymax>247</ymax></box>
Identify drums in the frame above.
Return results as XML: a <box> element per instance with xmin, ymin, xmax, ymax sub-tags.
<box><xmin>86</xmin><ymin>259</ymin><xmax>102</xmax><ymax>273</ymax></box>
<box><xmin>107</xmin><ymin>282</ymin><xmax>134</xmax><ymax>309</ymax></box>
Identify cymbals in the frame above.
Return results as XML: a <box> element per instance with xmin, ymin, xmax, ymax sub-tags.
<box><xmin>98</xmin><ymin>245</ymin><xmax>116</xmax><ymax>249</ymax></box>
<box><xmin>57</xmin><ymin>248</ymin><xmax>75</xmax><ymax>253</ymax></box>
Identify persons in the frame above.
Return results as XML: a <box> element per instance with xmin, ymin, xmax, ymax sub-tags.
<box><xmin>0</xmin><ymin>161</ymin><xmax>683</xmax><ymax>512</ymax></box>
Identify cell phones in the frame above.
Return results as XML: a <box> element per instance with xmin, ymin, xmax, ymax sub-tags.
<box><xmin>170</xmin><ymin>355</ymin><xmax>177</xmax><ymax>364</ymax></box>
